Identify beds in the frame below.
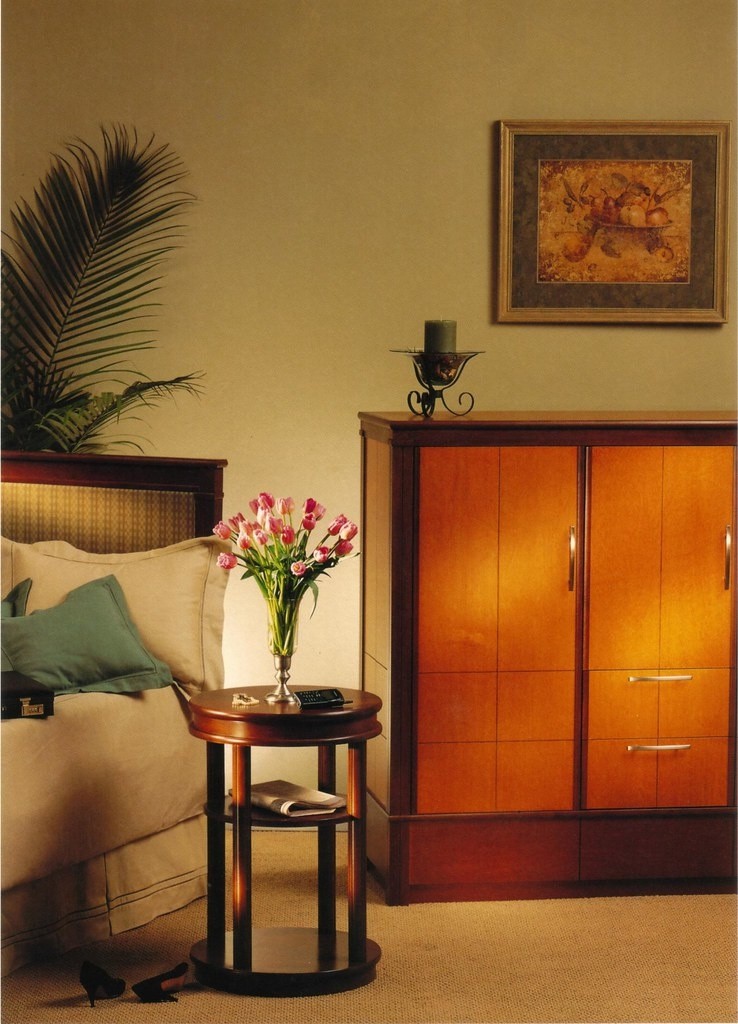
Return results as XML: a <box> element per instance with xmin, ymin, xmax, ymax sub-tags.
<box><xmin>0</xmin><ymin>446</ymin><xmax>225</xmax><ymax>979</ymax></box>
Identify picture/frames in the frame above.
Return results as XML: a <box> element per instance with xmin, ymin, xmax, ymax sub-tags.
<box><xmin>494</xmin><ymin>118</ymin><xmax>734</xmax><ymax>325</ymax></box>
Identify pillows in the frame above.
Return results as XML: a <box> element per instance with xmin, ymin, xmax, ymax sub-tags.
<box><xmin>10</xmin><ymin>535</ymin><xmax>234</xmax><ymax>694</ymax></box>
<box><xmin>1</xmin><ymin>572</ymin><xmax>175</xmax><ymax>699</ymax></box>
<box><xmin>1</xmin><ymin>578</ymin><xmax>34</xmax><ymax>674</ymax></box>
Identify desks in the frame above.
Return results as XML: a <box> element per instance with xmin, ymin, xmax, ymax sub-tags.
<box><xmin>185</xmin><ymin>683</ymin><xmax>383</xmax><ymax>988</ymax></box>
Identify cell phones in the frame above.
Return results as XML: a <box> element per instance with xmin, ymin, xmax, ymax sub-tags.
<box><xmin>293</xmin><ymin>689</ymin><xmax>354</xmax><ymax>710</ymax></box>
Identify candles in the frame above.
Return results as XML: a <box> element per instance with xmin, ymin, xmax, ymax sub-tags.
<box><xmin>422</xmin><ymin>311</ymin><xmax>458</xmax><ymax>354</ymax></box>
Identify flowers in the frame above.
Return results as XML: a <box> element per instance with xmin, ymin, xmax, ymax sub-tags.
<box><xmin>213</xmin><ymin>492</ymin><xmax>358</xmax><ymax>654</ymax></box>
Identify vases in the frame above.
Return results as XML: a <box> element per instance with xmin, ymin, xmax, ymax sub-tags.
<box><xmin>260</xmin><ymin>594</ymin><xmax>303</xmax><ymax>702</ymax></box>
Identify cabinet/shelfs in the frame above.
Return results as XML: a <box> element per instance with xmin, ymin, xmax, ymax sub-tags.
<box><xmin>356</xmin><ymin>408</ymin><xmax>738</xmax><ymax>821</ymax></box>
